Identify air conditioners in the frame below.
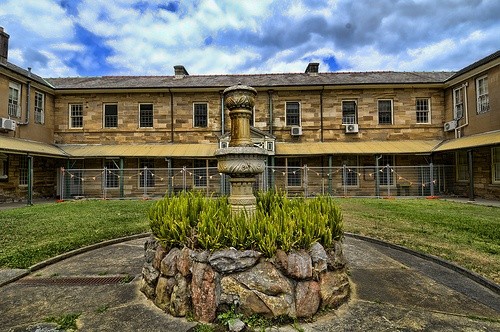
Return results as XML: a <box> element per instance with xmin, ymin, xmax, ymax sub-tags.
<box><xmin>345</xmin><ymin>123</ymin><xmax>358</xmax><ymax>134</ymax></box>
<box><xmin>290</xmin><ymin>126</ymin><xmax>303</xmax><ymax>135</ymax></box>
<box><xmin>444</xmin><ymin>120</ymin><xmax>457</xmax><ymax>131</ymax></box>
<box><xmin>0</xmin><ymin>117</ymin><xmax>17</xmax><ymax>132</ymax></box>
